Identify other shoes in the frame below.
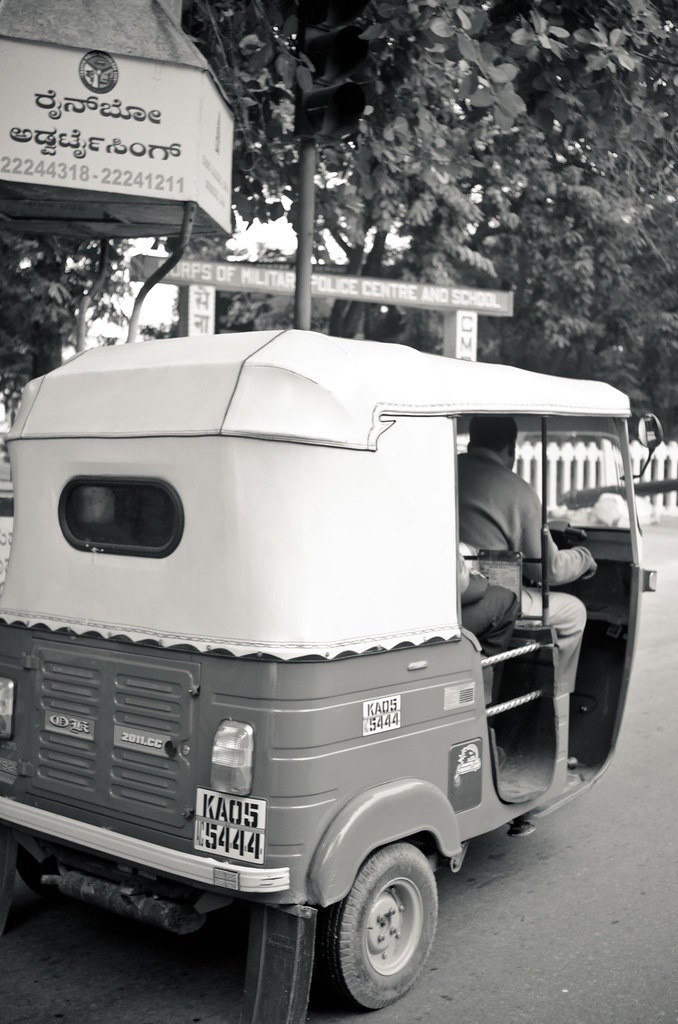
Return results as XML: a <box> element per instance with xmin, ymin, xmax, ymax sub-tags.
<box><xmin>497</xmin><ymin>745</ymin><xmax>507</xmax><ymax>768</ymax></box>
<box><xmin>570</xmin><ymin>691</ymin><xmax>597</xmax><ymax>716</ymax></box>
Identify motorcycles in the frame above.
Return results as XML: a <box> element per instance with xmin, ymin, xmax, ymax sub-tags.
<box><xmin>2</xmin><ymin>325</ymin><xmax>665</xmax><ymax>1010</ymax></box>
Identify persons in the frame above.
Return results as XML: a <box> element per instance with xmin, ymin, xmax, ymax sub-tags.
<box><xmin>457</xmin><ymin>417</ymin><xmax>597</xmax><ymax>713</ymax></box>
